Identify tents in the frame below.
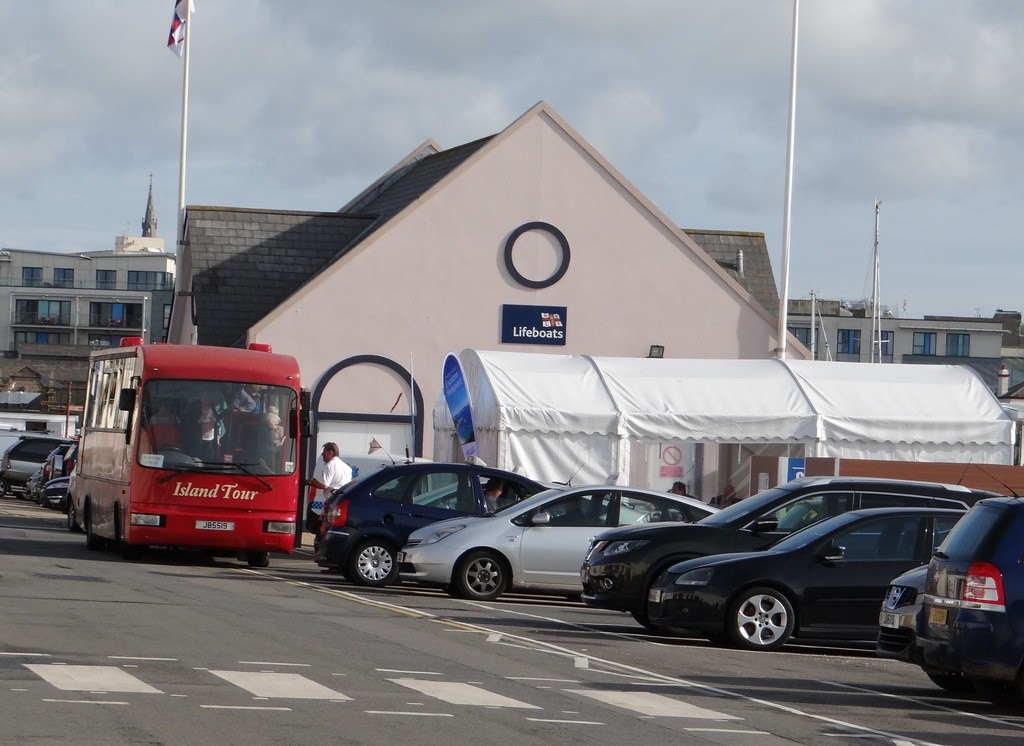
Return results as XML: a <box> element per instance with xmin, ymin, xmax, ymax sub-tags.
<box><xmin>430</xmin><ymin>349</ymin><xmax>1016</xmax><ymax>504</ymax></box>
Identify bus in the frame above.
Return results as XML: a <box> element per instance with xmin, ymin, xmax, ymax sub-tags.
<box><xmin>61</xmin><ymin>337</ymin><xmax>315</xmax><ymax>567</ymax></box>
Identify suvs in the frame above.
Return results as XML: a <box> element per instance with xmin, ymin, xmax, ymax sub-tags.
<box><xmin>578</xmin><ymin>475</ymin><xmax>1008</xmax><ymax>627</ymax></box>
<box><xmin>0</xmin><ymin>427</ymin><xmax>78</xmax><ymax>510</ymax></box>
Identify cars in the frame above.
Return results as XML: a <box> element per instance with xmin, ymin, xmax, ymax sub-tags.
<box><xmin>315</xmin><ymin>460</ymin><xmax>648</xmax><ymax>588</ymax></box>
<box><xmin>647</xmin><ymin>497</ymin><xmax>1022</xmax><ymax>713</ymax></box>
<box><xmin>396</xmin><ymin>484</ymin><xmax>721</xmax><ymax>600</ymax></box>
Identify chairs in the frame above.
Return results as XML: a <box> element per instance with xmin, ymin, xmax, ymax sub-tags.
<box><xmin>899</xmin><ymin>527</ymin><xmax>919</xmax><ymax>558</ymax></box>
<box><xmin>873</xmin><ymin>527</ymin><xmax>890</xmax><ymax>559</ymax></box>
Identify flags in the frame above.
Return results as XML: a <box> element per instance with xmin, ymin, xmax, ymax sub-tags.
<box><xmin>441</xmin><ymin>351</ymin><xmax>489</xmax><ymax>517</ymax></box>
<box><xmin>167</xmin><ymin>0</ymin><xmax>196</xmax><ymax>60</ymax></box>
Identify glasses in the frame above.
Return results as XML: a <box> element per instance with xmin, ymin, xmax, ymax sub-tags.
<box><xmin>498</xmin><ymin>488</ymin><xmax>503</xmax><ymax>494</ymax></box>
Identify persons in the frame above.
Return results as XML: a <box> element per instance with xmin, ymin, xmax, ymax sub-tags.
<box><xmin>710</xmin><ymin>485</ymin><xmax>744</xmax><ymax>509</ymax></box>
<box><xmin>671</xmin><ymin>482</ymin><xmax>696</xmax><ymax>500</ymax></box>
<box><xmin>483</xmin><ymin>476</ymin><xmax>503</xmax><ymax>512</ymax></box>
<box><xmin>149</xmin><ymin>379</ymin><xmax>286</xmax><ymax>475</ymax></box>
<box><xmin>306</xmin><ymin>442</ymin><xmax>353</xmax><ymax>576</ymax></box>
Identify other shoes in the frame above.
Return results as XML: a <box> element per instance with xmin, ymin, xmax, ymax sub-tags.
<box><xmin>321</xmin><ymin>568</ymin><xmax>338</xmax><ymax>575</ymax></box>
